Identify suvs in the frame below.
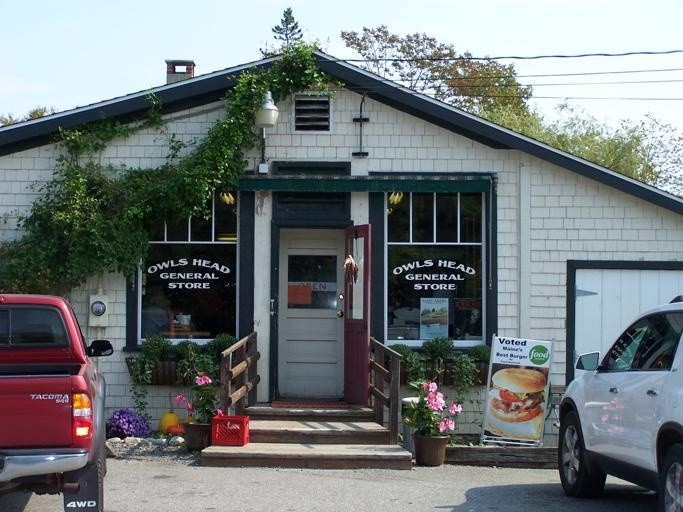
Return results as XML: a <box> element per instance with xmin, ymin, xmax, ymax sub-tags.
<box><xmin>387</xmin><ymin>305</ymin><xmax>420</xmax><ymax>340</ymax></box>
<box><xmin>553</xmin><ymin>295</ymin><xmax>682</xmax><ymax>511</ymax></box>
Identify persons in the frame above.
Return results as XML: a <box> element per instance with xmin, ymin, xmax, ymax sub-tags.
<box><xmin>456</xmin><ymin>308</ymin><xmax>481</xmax><ymax>339</ymax></box>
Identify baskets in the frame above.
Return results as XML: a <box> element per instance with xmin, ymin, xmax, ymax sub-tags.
<box><xmin>211</xmin><ymin>415</ymin><xmax>249</xmax><ymax>446</ymax></box>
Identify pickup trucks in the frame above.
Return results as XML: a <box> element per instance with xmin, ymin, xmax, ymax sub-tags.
<box><xmin>0</xmin><ymin>293</ymin><xmax>113</xmax><ymax>511</ymax></box>
<box><xmin>140</xmin><ymin>308</ymin><xmax>181</xmax><ymax>340</ymax></box>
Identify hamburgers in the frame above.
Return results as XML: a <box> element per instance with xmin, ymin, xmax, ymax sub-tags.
<box><xmin>489</xmin><ymin>367</ymin><xmax>547</xmax><ymax>424</ymax></box>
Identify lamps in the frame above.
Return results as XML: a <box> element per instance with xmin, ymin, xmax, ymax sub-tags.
<box><xmin>254</xmin><ymin>88</ymin><xmax>280</xmax><ymax>175</ymax></box>
<box><xmin>349</xmin><ymin>92</ymin><xmax>372</xmax><ymax>178</ymax></box>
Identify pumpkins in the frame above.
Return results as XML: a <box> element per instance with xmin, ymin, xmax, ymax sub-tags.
<box><xmin>160</xmin><ymin>413</ymin><xmax>179</xmax><ymax>435</ymax></box>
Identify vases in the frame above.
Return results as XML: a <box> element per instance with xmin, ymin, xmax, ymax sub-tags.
<box><xmin>411</xmin><ymin>433</ymin><xmax>452</xmax><ymax>467</ymax></box>
<box><xmin>181</xmin><ymin>421</ymin><xmax>211</xmax><ymax>452</ymax></box>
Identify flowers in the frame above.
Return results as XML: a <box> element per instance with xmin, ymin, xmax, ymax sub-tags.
<box><xmin>173</xmin><ymin>342</ymin><xmax>224</xmax><ymax>423</ymax></box>
<box><xmin>401</xmin><ymin>374</ymin><xmax>462</xmax><ymax>436</ymax></box>
